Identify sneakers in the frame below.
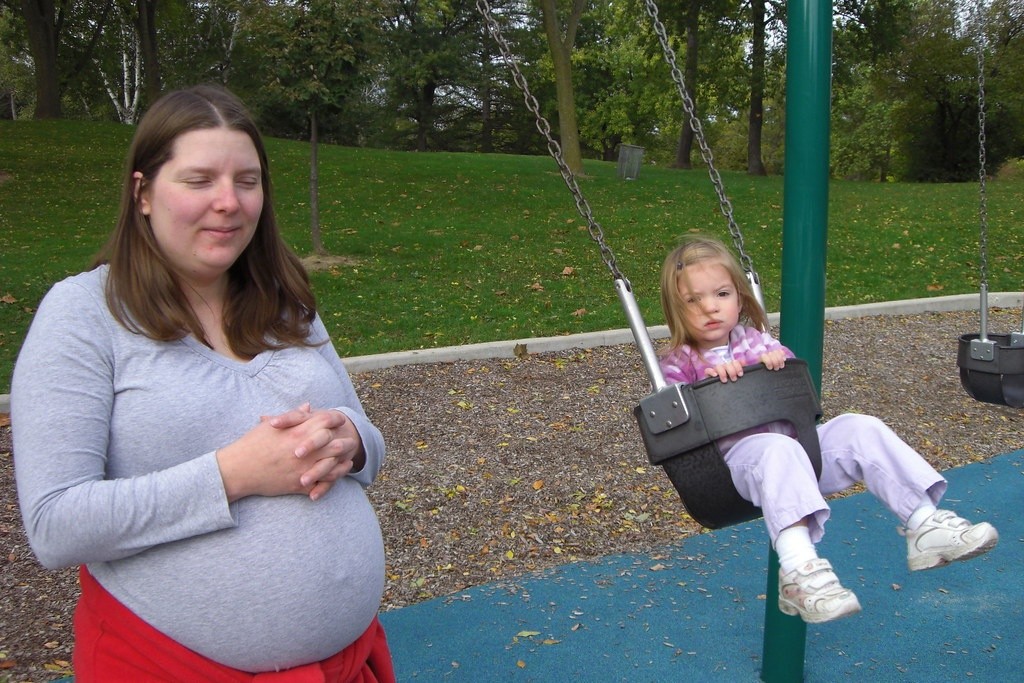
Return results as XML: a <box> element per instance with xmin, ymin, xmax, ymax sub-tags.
<box><xmin>905</xmin><ymin>509</ymin><xmax>998</xmax><ymax>571</ymax></box>
<box><xmin>779</xmin><ymin>558</ymin><xmax>861</xmax><ymax>623</ymax></box>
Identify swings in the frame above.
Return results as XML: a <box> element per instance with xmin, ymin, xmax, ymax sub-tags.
<box><xmin>956</xmin><ymin>0</ymin><xmax>1024</xmax><ymax>411</ymax></box>
<box><xmin>475</xmin><ymin>0</ymin><xmax>825</xmax><ymax>531</ymax></box>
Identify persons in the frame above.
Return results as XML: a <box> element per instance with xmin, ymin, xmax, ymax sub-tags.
<box><xmin>8</xmin><ymin>82</ymin><xmax>398</xmax><ymax>683</ymax></box>
<box><xmin>655</xmin><ymin>237</ymin><xmax>999</xmax><ymax>622</ymax></box>
